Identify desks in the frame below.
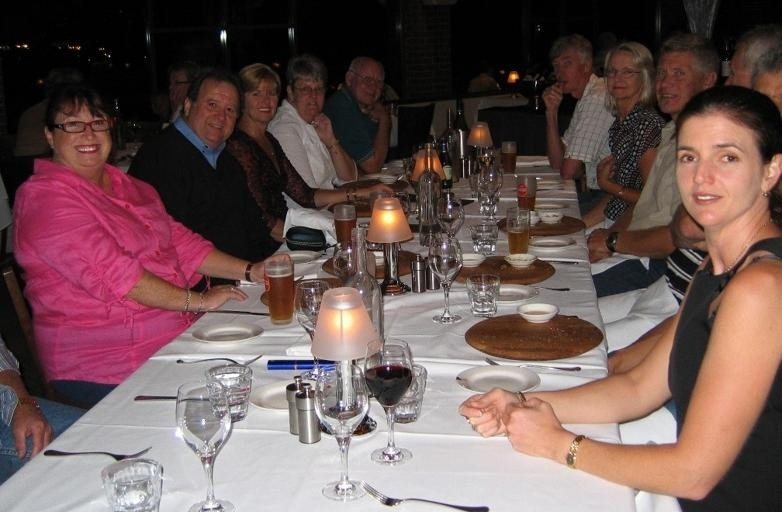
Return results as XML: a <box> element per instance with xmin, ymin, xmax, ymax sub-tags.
<box><xmin>478</xmin><ymin>104</ymin><xmax>573</xmax><ymax>156</ymax></box>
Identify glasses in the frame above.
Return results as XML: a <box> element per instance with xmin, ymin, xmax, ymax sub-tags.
<box><xmin>350</xmin><ymin>69</ymin><xmax>384</xmax><ymax>89</ymax></box>
<box><xmin>606</xmin><ymin>68</ymin><xmax>640</xmax><ymax>79</ymax></box>
<box><xmin>294</xmin><ymin>86</ymin><xmax>326</xmax><ymax>94</ymax></box>
<box><xmin>54</xmin><ymin>119</ymin><xmax>115</xmax><ymax>133</ymax></box>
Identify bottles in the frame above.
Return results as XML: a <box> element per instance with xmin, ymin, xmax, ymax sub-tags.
<box><xmin>112</xmin><ymin>98</ymin><xmax>127</xmax><ymax>148</ymax></box>
<box><xmin>284</xmin><ymin>374</ymin><xmax>323</xmax><ymax>444</ymax></box>
<box><xmin>454</xmin><ymin>96</ymin><xmax>468</xmax><ymax>159</ymax></box>
<box><xmin>410</xmin><ymin>256</ymin><xmax>441</xmax><ymax>292</ymax></box>
<box><xmin>720</xmin><ymin>42</ymin><xmax>731</xmax><ymax>80</ymax></box>
<box><xmin>346</xmin><ymin>225</ymin><xmax>387</xmax><ymax>338</ymax></box>
<box><xmin>532</xmin><ymin>80</ymin><xmax>540</xmax><ymax>112</ymax></box>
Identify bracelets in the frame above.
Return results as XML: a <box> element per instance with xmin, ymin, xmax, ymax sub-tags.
<box><xmin>243</xmin><ymin>261</ymin><xmax>256</xmax><ymax>281</ymax></box>
<box><xmin>614</xmin><ymin>186</ymin><xmax>623</xmax><ymax>198</ymax></box>
<box><xmin>196</xmin><ymin>288</ymin><xmax>206</xmax><ymax>314</ymax></box>
<box><xmin>328</xmin><ymin>140</ymin><xmax>341</xmax><ymax>151</ymax></box>
<box><xmin>14</xmin><ymin>395</ymin><xmax>43</xmax><ymax>410</ymax></box>
<box><xmin>514</xmin><ymin>389</ymin><xmax>528</xmax><ymax>404</ymax></box>
<box><xmin>180</xmin><ymin>287</ymin><xmax>194</xmax><ymax>313</ymax></box>
<box><xmin>565</xmin><ymin>433</ymin><xmax>584</xmax><ymax>464</ymax></box>
<box><xmin>344</xmin><ymin>185</ymin><xmax>359</xmax><ymax>203</ymax></box>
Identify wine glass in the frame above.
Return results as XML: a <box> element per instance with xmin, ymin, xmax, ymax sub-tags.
<box><xmin>316</xmin><ymin>364</ymin><xmax>370</xmax><ymax>503</ymax></box>
<box><xmin>177</xmin><ymin>382</ymin><xmax>237</xmax><ymax>512</ymax></box>
<box><xmin>365</xmin><ymin>341</ymin><xmax>412</xmax><ymax>467</ymax></box>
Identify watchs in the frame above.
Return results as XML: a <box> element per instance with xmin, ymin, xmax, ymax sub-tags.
<box><xmin>604</xmin><ymin>230</ymin><xmax>619</xmax><ymax>253</ymax></box>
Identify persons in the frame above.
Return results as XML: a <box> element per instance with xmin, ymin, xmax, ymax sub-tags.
<box><xmin>321</xmin><ymin>52</ymin><xmax>393</xmax><ymax>177</ymax></box>
<box><xmin>160</xmin><ymin>61</ymin><xmax>202</xmax><ymax>135</ymax></box>
<box><xmin>540</xmin><ymin>29</ymin><xmax>622</xmax><ymax>196</ymax></box>
<box><xmin>0</xmin><ymin>335</ymin><xmax>92</xmax><ymax>488</ymax></box>
<box><xmin>581</xmin><ymin>38</ymin><xmax>665</xmax><ymax>240</ymax></box>
<box><xmin>600</xmin><ymin>40</ymin><xmax>782</xmax><ymax>449</ymax></box>
<box><xmin>12</xmin><ymin>65</ymin><xmax>91</xmax><ymax>159</ymax></box>
<box><xmin>0</xmin><ymin>171</ymin><xmax>16</xmax><ymax>250</ymax></box>
<box><xmin>119</xmin><ymin>61</ymin><xmax>288</xmax><ymax>292</ymax></box>
<box><xmin>581</xmin><ymin>31</ymin><xmax>725</xmax><ymax>301</ymax></box>
<box><xmin>455</xmin><ymin>83</ymin><xmax>781</xmax><ymax>512</ymax></box>
<box><xmin>10</xmin><ymin>81</ymin><xmax>293</xmax><ymax>413</ymax></box>
<box><xmin>265</xmin><ymin>48</ymin><xmax>357</xmax><ymax>201</ymax></box>
<box><xmin>219</xmin><ymin>59</ymin><xmax>394</xmax><ymax>261</ymax></box>
<box><xmin>591</xmin><ymin>22</ymin><xmax>782</xmax><ymax>355</ymax></box>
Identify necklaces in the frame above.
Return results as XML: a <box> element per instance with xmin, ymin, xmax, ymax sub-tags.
<box><xmin>718</xmin><ymin>219</ymin><xmax>771</xmax><ymax>275</ymax></box>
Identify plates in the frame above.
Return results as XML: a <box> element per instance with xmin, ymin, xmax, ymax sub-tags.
<box><xmin>494</xmin><ymin>284</ymin><xmax>539</xmax><ymax>306</ymax></box>
<box><xmin>535</xmin><ymin>202</ymin><xmax>570</xmax><ymax>211</ymax></box>
<box><xmin>537</xmin><ymin>181</ymin><xmax>558</xmax><ymax>190</ymax></box>
<box><xmin>280</xmin><ymin>250</ymin><xmax>323</xmax><ymax>264</ymax></box>
<box><xmin>528</xmin><ymin>236</ymin><xmax>576</xmax><ymax>249</ymax></box>
<box><xmin>249</xmin><ymin>380</ymin><xmax>319</xmax><ymax>410</ymax></box>
<box><xmin>457</xmin><ymin>364</ymin><xmax>542</xmax><ymax>397</ymax></box>
<box><xmin>191</xmin><ymin>321</ymin><xmax>265</xmax><ymax>342</ymax></box>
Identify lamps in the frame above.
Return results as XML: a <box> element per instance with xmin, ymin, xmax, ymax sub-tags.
<box><xmin>507</xmin><ymin>71</ymin><xmax>520</xmax><ymax>98</ymax></box>
<box><xmin>412</xmin><ymin>149</ymin><xmax>446</xmax><ymax>219</ymax></box>
<box><xmin>309</xmin><ymin>287</ymin><xmax>383</xmax><ymax>436</ymax></box>
<box><xmin>366</xmin><ymin>197</ymin><xmax>414</xmax><ymax>296</ymax></box>
<box><xmin>468</xmin><ymin>122</ymin><xmax>494</xmax><ymax>173</ymax></box>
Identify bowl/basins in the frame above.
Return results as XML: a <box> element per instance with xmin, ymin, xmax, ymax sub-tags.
<box><xmin>541</xmin><ymin>212</ymin><xmax>564</xmax><ymax>225</ymax></box>
<box><xmin>519</xmin><ymin>302</ymin><xmax>558</xmax><ymax>323</ymax></box>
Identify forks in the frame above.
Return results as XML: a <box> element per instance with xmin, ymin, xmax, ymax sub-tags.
<box><xmin>176</xmin><ymin>355</ymin><xmax>262</xmax><ymax>367</ymax></box>
<box><xmin>359</xmin><ymin>480</ymin><xmax>490</xmax><ymax>512</ymax></box>
<box><xmin>43</xmin><ymin>447</ymin><xmax>155</xmax><ymax>461</ymax></box>
<box><xmin>484</xmin><ymin>357</ymin><xmax>582</xmax><ymax>372</ymax></box>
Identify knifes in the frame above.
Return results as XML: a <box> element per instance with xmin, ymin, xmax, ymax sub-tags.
<box><xmin>543</xmin><ymin>260</ymin><xmax>580</xmax><ymax>266</ymax></box>
<box><xmin>132</xmin><ymin>394</ymin><xmax>209</xmax><ymax>401</ymax></box>
<box><xmin>209</xmin><ymin>309</ymin><xmax>270</xmax><ymax>316</ymax></box>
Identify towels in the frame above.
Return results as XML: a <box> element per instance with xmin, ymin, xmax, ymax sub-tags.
<box><xmin>590</xmin><ymin>252</ymin><xmax>650</xmax><ymax>275</ymax></box>
<box><xmin>286</xmin><ymin>332</ymin><xmax>313</xmax><ymax>356</ymax></box>
<box><xmin>516</xmin><ymin>155</ymin><xmax>550</xmax><ymax>167</ymax></box>
<box><xmin>280</xmin><ymin>190</ymin><xmax>338</xmax><ymax>246</ymax></box>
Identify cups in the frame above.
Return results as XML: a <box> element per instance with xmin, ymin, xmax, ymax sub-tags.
<box><xmin>394</xmin><ymin>364</ymin><xmax>426</xmax><ymax>423</ymax></box>
<box><xmin>322</xmin><ymin>141</ymin><xmax>538</xmax><ymax>276</ymax></box>
<box><xmin>468</xmin><ymin>274</ymin><xmax>501</xmax><ymax>316</ymax></box>
<box><xmin>388</xmin><ymin>103</ymin><xmax>398</xmax><ymax>117</ymax></box>
<box><xmin>128</xmin><ymin>118</ymin><xmax>143</xmax><ymax>137</ymax></box>
<box><xmin>294</xmin><ymin>279</ymin><xmax>330</xmax><ymax>344</ymax></box>
<box><xmin>102</xmin><ymin>456</ymin><xmax>164</xmax><ymax>512</ymax></box>
<box><xmin>264</xmin><ymin>257</ymin><xmax>294</xmax><ymax>325</ymax></box>
<box><xmin>205</xmin><ymin>363</ymin><xmax>254</xmax><ymax>422</ymax></box>
<box><xmin>427</xmin><ymin>239</ymin><xmax>464</xmax><ymax>325</ymax></box>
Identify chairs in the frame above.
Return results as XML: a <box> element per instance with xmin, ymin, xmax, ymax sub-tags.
<box><xmin>1</xmin><ymin>251</ymin><xmax>93</xmax><ymax>411</ymax></box>
<box><xmin>396</xmin><ymin>102</ymin><xmax>435</xmax><ymax>159</ymax></box>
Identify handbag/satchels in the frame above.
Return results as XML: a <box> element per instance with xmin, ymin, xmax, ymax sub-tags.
<box><xmin>285</xmin><ymin>226</ymin><xmax>325</xmax><ymax>251</ymax></box>
